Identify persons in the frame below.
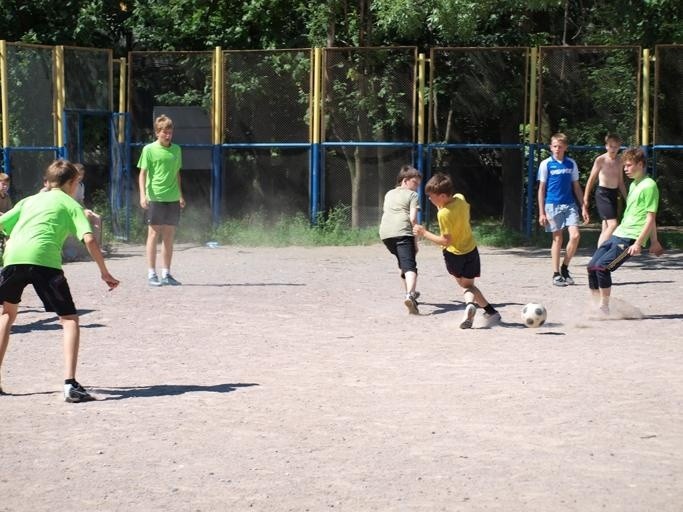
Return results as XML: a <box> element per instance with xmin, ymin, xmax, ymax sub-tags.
<box><xmin>0</xmin><ymin>160</ymin><xmax>120</xmax><ymax>403</ymax></box>
<box><xmin>379</xmin><ymin>164</ymin><xmax>423</xmax><ymax>315</ymax></box>
<box><xmin>536</xmin><ymin>133</ymin><xmax>589</xmax><ymax>286</ymax></box>
<box><xmin>0</xmin><ymin>162</ymin><xmax>109</xmax><ymax>273</ymax></box>
<box><xmin>584</xmin><ymin>134</ymin><xmax>629</xmax><ymax>249</ymax></box>
<box><xmin>586</xmin><ymin>147</ymin><xmax>663</xmax><ymax>322</ymax></box>
<box><xmin>412</xmin><ymin>172</ymin><xmax>501</xmax><ymax>330</ymax></box>
<box><xmin>136</xmin><ymin>114</ymin><xmax>186</xmax><ymax>286</ymax></box>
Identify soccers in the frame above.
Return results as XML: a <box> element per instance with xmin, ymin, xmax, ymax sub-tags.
<box><xmin>521</xmin><ymin>304</ymin><xmax>545</xmax><ymax>328</ymax></box>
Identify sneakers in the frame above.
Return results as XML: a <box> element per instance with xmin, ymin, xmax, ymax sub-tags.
<box><xmin>460</xmin><ymin>304</ymin><xmax>477</xmax><ymax>328</ymax></box>
<box><xmin>553</xmin><ymin>276</ymin><xmax>574</xmax><ymax>286</ymax></box>
<box><xmin>63</xmin><ymin>382</ymin><xmax>91</xmax><ymax>402</ymax></box>
<box><xmin>481</xmin><ymin>312</ymin><xmax>501</xmax><ymax>328</ymax></box>
<box><xmin>150</xmin><ymin>274</ymin><xmax>181</xmax><ymax>286</ymax></box>
<box><xmin>404</xmin><ymin>293</ymin><xmax>420</xmax><ymax>314</ymax></box>
